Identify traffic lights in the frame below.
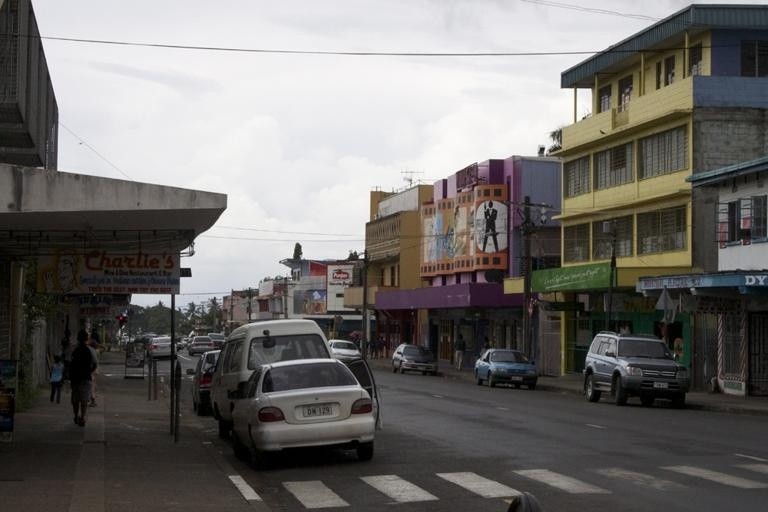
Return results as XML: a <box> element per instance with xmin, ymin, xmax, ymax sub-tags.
<box><xmin>115</xmin><ymin>314</ymin><xmax>129</xmax><ymax>328</ymax></box>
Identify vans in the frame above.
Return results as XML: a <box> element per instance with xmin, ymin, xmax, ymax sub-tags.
<box><xmin>209</xmin><ymin>318</ymin><xmax>339</xmax><ymax>440</ymax></box>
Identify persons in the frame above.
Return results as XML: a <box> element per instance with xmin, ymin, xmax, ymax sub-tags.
<box><xmin>343</xmin><ymin>334</ymin><xmax>392</xmax><ymax>360</ymax></box>
<box><xmin>482</xmin><ymin>201</ymin><xmax>499</xmax><ymax>253</ymax></box>
<box><xmin>49</xmin><ymin>328</ymin><xmax>107</xmax><ymax>428</ymax></box>
<box><xmin>452</xmin><ymin>333</ymin><xmax>466</xmax><ymax>371</ymax></box>
<box><xmin>474</xmin><ymin>331</ymin><xmax>492</xmax><ymax>359</ymax></box>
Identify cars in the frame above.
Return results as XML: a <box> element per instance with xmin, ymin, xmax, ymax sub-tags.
<box><xmin>329</xmin><ymin>339</ymin><xmax>362</xmax><ymax>363</ymax></box>
<box><xmin>227</xmin><ymin>358</ymin><xmax>383</xmax><ymax>462</ymax></box>
<box><xmin>118</xmin><ymin>332</ymin><xmax>270</xmax><ymax>417</ymax></box>
<box><xmin>474</xmin><ymin>348</ymin><xmax>538</xmax><ymax>391</ymax></box>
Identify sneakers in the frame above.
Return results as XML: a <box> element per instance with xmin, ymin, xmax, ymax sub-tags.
<box><xmin>73</xmin><ymin>416</ymin><xmax>85</xmax><ymax>427</ymax></box>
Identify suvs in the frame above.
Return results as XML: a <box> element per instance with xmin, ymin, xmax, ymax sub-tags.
<box><xmin>392</xmin><ymin>342</ymin><xmax>438</xmax><ymax>376</ymax></box>
<box><xmin>582</xmin><ymin>330</ymin><xmax>690</xmax><ymax>406</ymax></box>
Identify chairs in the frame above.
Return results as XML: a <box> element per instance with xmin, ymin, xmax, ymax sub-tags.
<box><xmin>279</xmin><ymin>349</ymin><xmax>295</xmax><ymax>361</ymax></box>
